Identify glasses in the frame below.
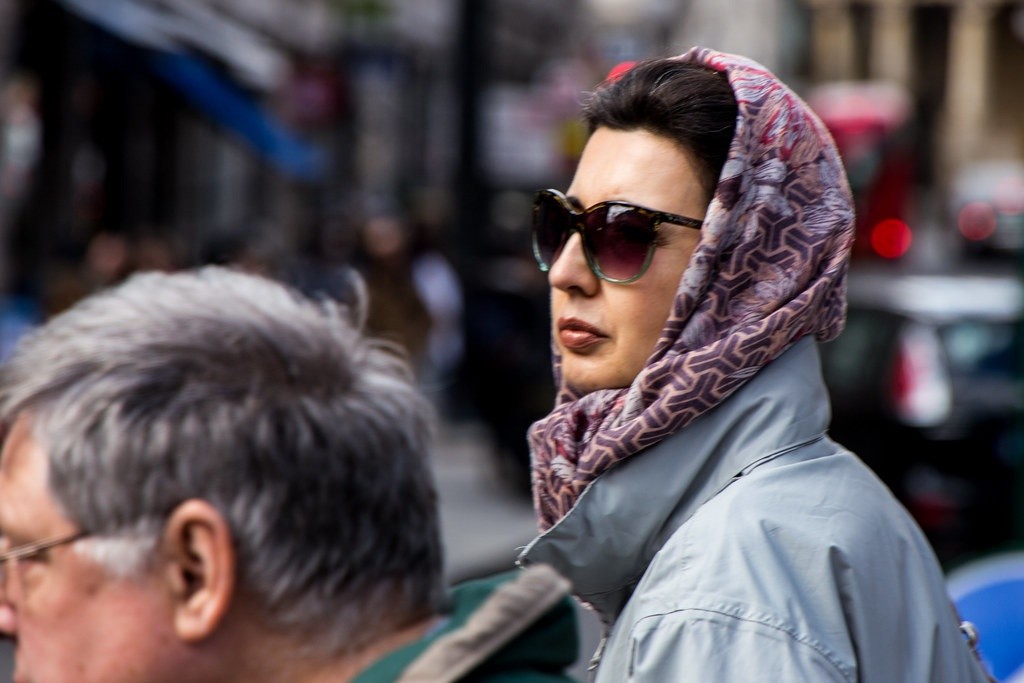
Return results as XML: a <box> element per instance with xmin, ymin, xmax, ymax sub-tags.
<box><xmin>0</xmin><ymin>504</ymin><xmax>172</xmax><ymax>610</ymax></box>
<box><xmin>528</xmin><ymin>187</ymin><xmax>705</xmax><ymax>284</ymax></box>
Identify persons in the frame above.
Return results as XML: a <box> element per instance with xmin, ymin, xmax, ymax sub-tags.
<box><xmin>1</xmin><ymin>260</ymin><xmax>572</xmax><ymax>683</ymax></box>
<box><xmin>518</xmin><ymin>48</ymin><xmax>995</xmax><ymax>683</ymax></box>
<box><xmin>0</xmin><ymin>176</ymin><xmax>554</xmax><ymax>497</ymax></box>
